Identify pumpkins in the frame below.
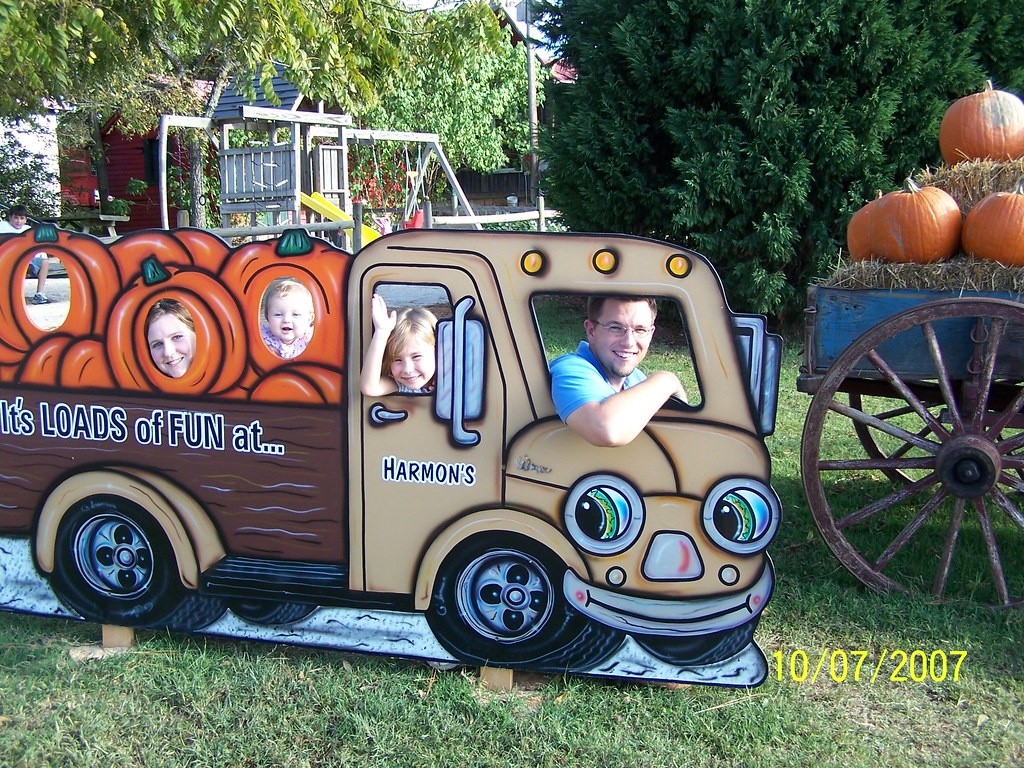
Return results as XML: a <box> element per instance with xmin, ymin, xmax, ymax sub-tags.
<box><xmin>847</xmin><ymin>188</ymin><xmax>883</xmax><ymax>262</ymax></box>
<box><xmin>867</xmin><ymin>178</ymin><xmax>962</xmax><ymax>264</ymax></box>
<box><xmin>961</xmin><ymin>176</ymin><xmax>1024</xmax><ymax>268</ymax></box>
<box><xmin>939</xmin><ymin>80</ymin><xmax>1024</xmax><ymax>166</ymax></box>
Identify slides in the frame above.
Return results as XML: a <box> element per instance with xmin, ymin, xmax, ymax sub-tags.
<box><xmin>301</xmin><ymin>190</ymin><xmax>382</xmax><ymax>246</ymax></box>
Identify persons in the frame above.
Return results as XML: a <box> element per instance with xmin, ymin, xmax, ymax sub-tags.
<box><xmin>144</xmin><ymin>299</ymin><xmax>196</xmax><ymax>378</ymax></box>
<box><xmin>0</xmin><ymin>206</ymin><xmax>50</xmax><ymax>304</ymax></box>
<box><xmin>359</xmin><ymin>294</ymin><xmax>438</xmax><ymax>398</ymax></box>
<box><xmin>546</xmin><ymin>296</ymin><xmax>688</xmax><ymax>447</ymax></box>
<box><xmin>261</xmin><ymin>281</ymin><xmax>313</xmax><ymax>359</ymax></box>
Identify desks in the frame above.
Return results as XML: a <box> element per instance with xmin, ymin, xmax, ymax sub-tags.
<box><xmin>34</xmin><ymin>216</ymin><xmax>131</xmax><ymax>245</ymax></box>
